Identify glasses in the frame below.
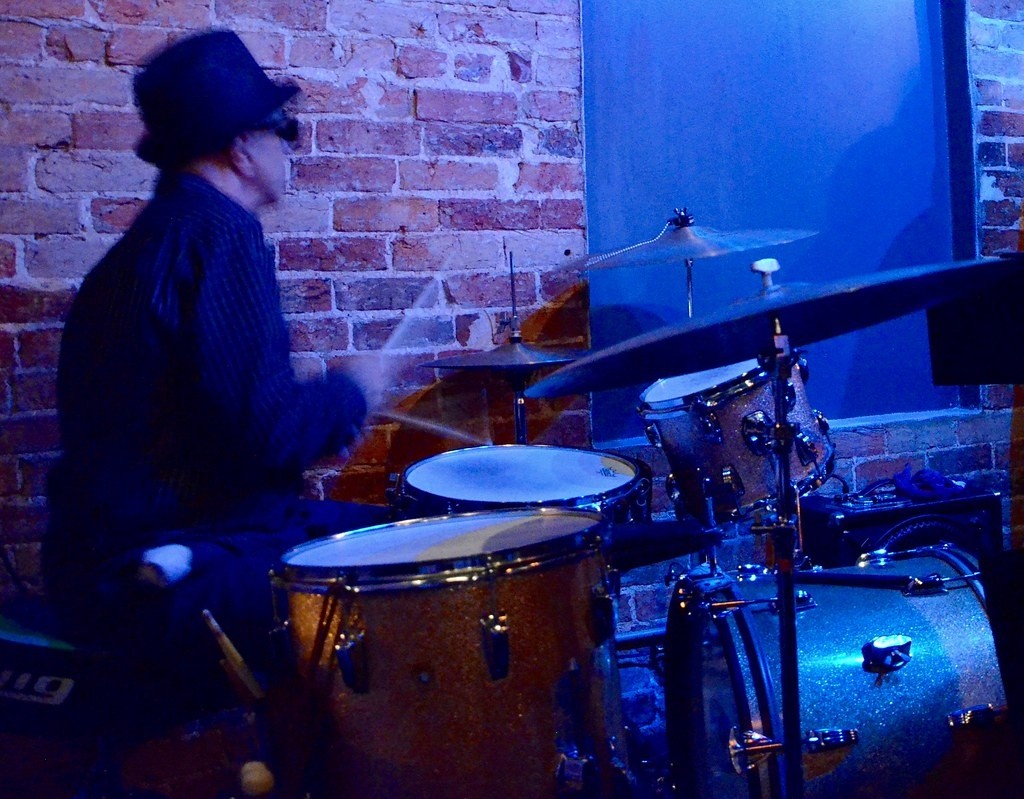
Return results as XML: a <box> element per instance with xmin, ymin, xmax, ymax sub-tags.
<box><xmin>251</xmin><ymin>116</ymin><xmax>299</xmax><ymax>142</ymax></box>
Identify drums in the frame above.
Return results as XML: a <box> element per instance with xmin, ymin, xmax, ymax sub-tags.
<box><xmin>387</xmin><ymin>441</ymin><xmax>654</xmax><ymax>526</ymax></box>
<box><xmin>274</xmin><ymin>509</ymin><xmax>632</xmax><ymax>799</ymax></box>
<box><xmin>636</xmin><ymin>348</ymin><xmax>838</xmax><ymax>520</ymax></box>
<box><xmin>660</xmin><ymin>545</ymin><xmax>1024</xmax><ymax>799</ymax></box>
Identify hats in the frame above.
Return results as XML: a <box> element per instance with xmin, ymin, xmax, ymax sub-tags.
<box><xmin>132</xmin><ymin>30</ymin><xmax>301</xmax><ymax>161</ymax></box>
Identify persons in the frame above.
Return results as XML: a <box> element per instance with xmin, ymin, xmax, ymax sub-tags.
<box><xmin>59</xmin><ymin>29</ymin><xmax>395</xmax><ymax>666</ymax></box>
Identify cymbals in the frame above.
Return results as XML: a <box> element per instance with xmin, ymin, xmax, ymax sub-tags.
<box><xmin>558</xmin><ymin>224</ymin><xmax>819</xmax><ymax>269</ymax></box>
<box><xmin>523</xmin><ymin>257</ymin><xmax>1009</xmax><ymax>397</ymax></box>
<box><xmin>416</xmin><ymin>343</ymin><xmax>577</xmax><ymax>368</ymax></box>
